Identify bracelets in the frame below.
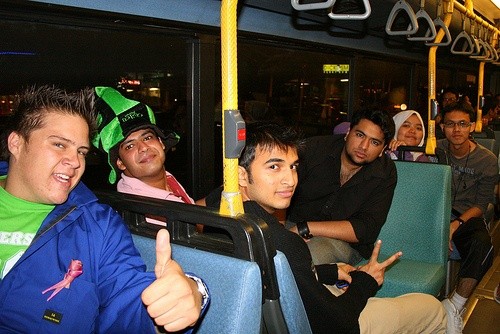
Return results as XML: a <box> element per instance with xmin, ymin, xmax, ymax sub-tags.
<box><xmin>296</xmin><ymin>221</ymin><xmax>314</xmax><ymax>240</ymax></box>
<box><xmin>456</xmin><ymin>218</ymin><xmax>465</xmax><ymax>224</ymax></box>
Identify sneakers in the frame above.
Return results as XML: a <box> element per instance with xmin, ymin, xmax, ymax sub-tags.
<box><xmin>441</xmin><ymin>298</ymin><xmax>467</xmax><ymax>334</ymax></box>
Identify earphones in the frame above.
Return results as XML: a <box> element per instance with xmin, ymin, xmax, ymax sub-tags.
<box><xmin>463</xmin><ymin>184</ymin><xmax>466</xmax><ymax>188</ymax></box>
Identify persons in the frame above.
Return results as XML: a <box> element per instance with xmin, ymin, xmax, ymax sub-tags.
<box><xmin>0</xmin><ymin>83</ymin><xmax>211</xmax><ymax>334</ymax></box>
<box><xmin>195</xmin><ymin>122</ymin><xmax>448</xmax><ymax>334</ymax></box>
<box><xmin>88</xmin><ymin>86</ymin><xmax>195</xmax><ymax>233</ymax></box>
<box><xmin>283</xmin><ymin>89</ymin><xmax>500</xmax><ymax>334</ymax></box>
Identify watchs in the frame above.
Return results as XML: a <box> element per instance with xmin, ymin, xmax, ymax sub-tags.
<box><xmin>184</xmin><ymin>274</ymin><xmax>210</xmax><ymax>310</ymax></box>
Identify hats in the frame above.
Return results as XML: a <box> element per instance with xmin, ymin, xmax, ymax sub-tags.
<box><xmin>91</xmin><ymin>86</ymin><xmax>179</xmax><ymax>185</ymax></box>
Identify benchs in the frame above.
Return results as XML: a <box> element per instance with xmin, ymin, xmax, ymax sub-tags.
<box><xmin>90</xmin><ymin>131</ymin><xmax>500</xmax><ymax>334</ymax></box>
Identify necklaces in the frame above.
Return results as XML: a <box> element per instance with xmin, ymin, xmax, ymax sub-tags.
<box><xmin>448</xmin><ymin>140</ymin><xmax>470</xmax><ymax>205</ymax></box>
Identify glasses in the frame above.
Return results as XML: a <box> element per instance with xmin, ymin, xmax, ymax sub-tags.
<box><xmin>444</xmin><ymin>120</ymin><xmax>473</xmax><ymax>128</ymax></box>
